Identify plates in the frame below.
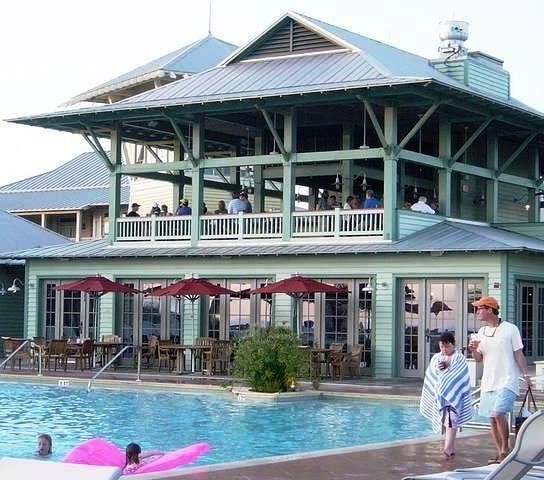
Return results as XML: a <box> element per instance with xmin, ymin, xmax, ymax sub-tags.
<box><xmin>297</xmin><ymin>345</ymin><xmax>310</xmax><ymax>349</ymax></box>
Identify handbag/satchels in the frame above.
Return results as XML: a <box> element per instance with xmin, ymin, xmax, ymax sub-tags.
<box><xmin>516</xmin><ymin>408</ymin><xmax>534</xmax><ymax>439</ymax></box>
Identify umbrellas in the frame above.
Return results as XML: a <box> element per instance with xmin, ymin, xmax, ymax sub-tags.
<box><xmin>49</xmin><ymin>274</ymin><xmax>139</xmax><ymax>371</ymax></box>
<box><xmin>242</xmin><ymin>277</ymin><xmax>353</xmax><ymax>380</ymax></box>
<box><xmin>140</xmin><ymin>276</ymin><xmax>239</xmax><ymax>375</ymax></box>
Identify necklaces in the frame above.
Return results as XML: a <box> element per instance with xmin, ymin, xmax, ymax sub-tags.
<box><xmin>481</xmin><ymin>318</ymin><xmax>502</xmax><ymax>338</ymax></box>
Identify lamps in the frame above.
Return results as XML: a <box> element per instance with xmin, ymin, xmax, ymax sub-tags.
<box><xmin>362</xmin><ymin>277</ymin><xmax>375</xmax><ymax>293</ymax></box>
<box><xmin>514</xmin><ymin>194</ymin><xmax>531</xmax><ymax>211</ymax></box>
<box><xmin>412</xmin><ymin>181</ymin><xmax>419</xmax><ymax>199</ymax></box>
<box><xmin>8</xmin><ymin>278</ymin><xmax>24</xmax><ymax>293</ymax></box>
<box><xmin>433</xmin><ymin>186</ymin><xmax>437</xmax><ymax>199</ymax></box>
<box><xmin>360</xmin><ymin>170</ymin><xmax>369</xmax><ymax>191</ymax></box>
<box><xmin>331</xmin><ymin>171</ymin><xmax>343</xmax><ymax>188</ymax></box>
<box><xmin>240</xmin><ymin>179</ymin><xmax>252</xmax><ymax>194</ymax></box>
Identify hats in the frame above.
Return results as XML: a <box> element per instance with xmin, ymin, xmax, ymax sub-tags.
<box><xmin>471</xmin><ymin>297</ymin><xmax>499</xmax><ymax>310</ymax></box>
<box><xmin>132</xmin><ymin>203</ymin><xmax>141</xmax><ymax>207</ymax></box>
<box><xmin>180</xmin><ymin>199</ymin><xmax>188</xmax><ymax>205</ymax></box>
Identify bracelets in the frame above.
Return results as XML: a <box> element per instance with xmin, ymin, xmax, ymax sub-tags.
<box><xmin>523</xmin><ymin>374</ymin><xmax>530</xmax><ymax>378</ymax></box>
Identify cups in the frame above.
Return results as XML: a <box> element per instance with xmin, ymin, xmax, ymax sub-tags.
<box><xmin>439</xmin><ymin>360</ymin><xmax>448</xmax><ymax>370</ymax></box>
<box><xmin>67</xmin><ymin>337</ymin><xmax>91</xmax><ymax>344</ymax></box>
<box><xmin>470</xmin><ymin>333</ymin><xmax>481</xmax><ymax>349</ymax></box>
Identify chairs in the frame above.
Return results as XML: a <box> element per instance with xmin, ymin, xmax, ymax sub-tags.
<box><xmin>402</xmin><ymin>410</ymin><xmax>544</xmax><ymax>480</ymax></box>
<box><xmin>1</xmin><ymin>334</ymin><xmax>233</xmax><ymax>376</ymax></box>
<box><xmin>295</xmin><ymin>343</ymin><xmax>364</xmax><ymax>382</ymax></box>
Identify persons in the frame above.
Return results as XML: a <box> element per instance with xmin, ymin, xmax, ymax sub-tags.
<box><xmin>317</xmin><ymin>190</ymin><xmax>384</xmax><ymax>231</ymax></box>
<box><xmin>32</xmin><ymin>435</ymin><xmax>53</xmax><ymax>456</ymax></box>
<box><xmin>118</xmin><ymin>441</ymin><xmax>165</xmax><ymax>475</ymax></box>
<box><xmin>465</xmin><ymin>296</ymin><xmax>537</xmax><ymax>466</ymax></box>
<box><xmin>203</xmin><ymin>192</ymin><xmax>252</xmax><ymax>234</ymax></box>
<box><xmin>127</xmin><ymin>199</ymin><xmax>192</xmax><ymax>236</ymax></box>
<box><xmin>418</xmin><ymin>331</ymin><xmax>474</xmax><ymax>460</ymax></box>
<box><xmin>401</xmin><ymin>196</ymin><xmax>439</xmax><ymax>215</ymax></box>
